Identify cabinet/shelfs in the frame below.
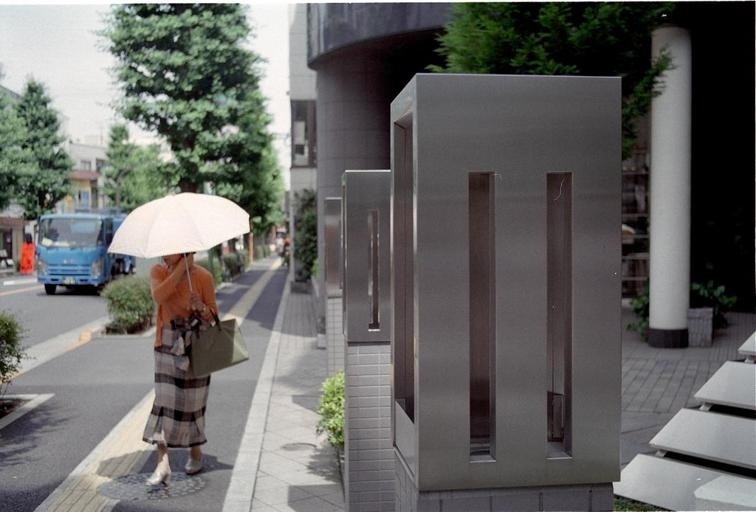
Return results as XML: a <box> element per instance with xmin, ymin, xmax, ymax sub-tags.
<box><xmin>621</xmin><ymin>149</ymin><xmax>650</xmax><ymax>285</ymax></box>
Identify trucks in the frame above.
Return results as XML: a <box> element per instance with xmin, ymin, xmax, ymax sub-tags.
<box><xmin>35</xmin><ymin>211</ymin><xmax>136</xmax><ymax>296</ymax></box>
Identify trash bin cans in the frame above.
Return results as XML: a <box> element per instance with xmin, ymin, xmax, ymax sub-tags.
<box><xmin>0</xmin><ymin>249</ymin><xmax>8</xmax><ymax>269</ymax></box>
<box><xmin>688</xmin><ymin>308</ymin><xmax>713</xmax><ymax>348</ymax></box>
<box><xmin>257</xmin><ymin>245</ymin><xmax>271</xmax><ymax>257</ymax></box>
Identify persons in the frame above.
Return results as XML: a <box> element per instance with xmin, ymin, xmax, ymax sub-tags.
<box><xmin>146</xmin><ymin>250</ymin><xmax>216</xmax><ymax>486</ymax></box>
<box><xmin>19</xmin><ymin>234</ymin><xmax>34</xmax><ymax>273</ymax></box>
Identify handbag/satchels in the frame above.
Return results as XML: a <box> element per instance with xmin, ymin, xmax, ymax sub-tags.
<box><xmin>189</xmin><ymin>312</ymin><xmax>248</xmax><ymax>379</ymax></box>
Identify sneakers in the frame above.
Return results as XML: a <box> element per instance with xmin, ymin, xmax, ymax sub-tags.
<box><xmin>185</xmin><ymin>457</ymin><xmax>205</xmax><ymax>475</ymax></box>
<box><xmin>144</xmin><ymin>466</ymin><xmax>171</xmax><ymax>486</ymax></box>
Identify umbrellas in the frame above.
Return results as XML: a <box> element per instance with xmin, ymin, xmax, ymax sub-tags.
<box><xmin>108</xmin><ymin>191</ymin><xmax>251</xmax><ymax>311</ymax></box>
